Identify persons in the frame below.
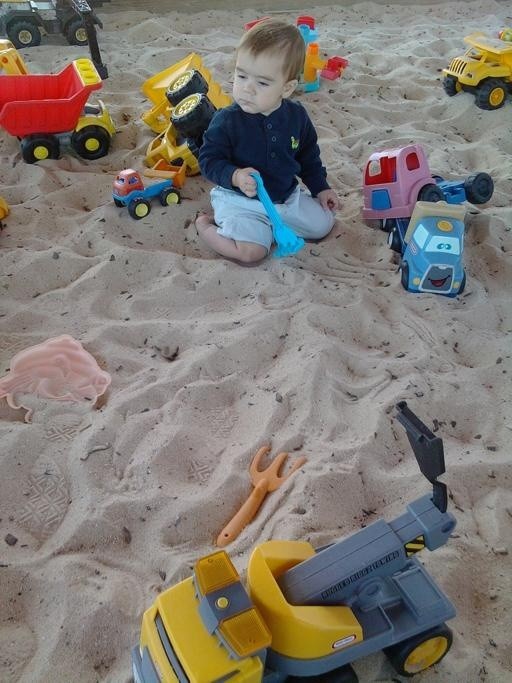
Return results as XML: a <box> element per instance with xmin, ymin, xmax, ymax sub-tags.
<box><xmin>194</xmin><ymin>18</ymin><xmax>345</xmax><ymax>264</ymax></box>
